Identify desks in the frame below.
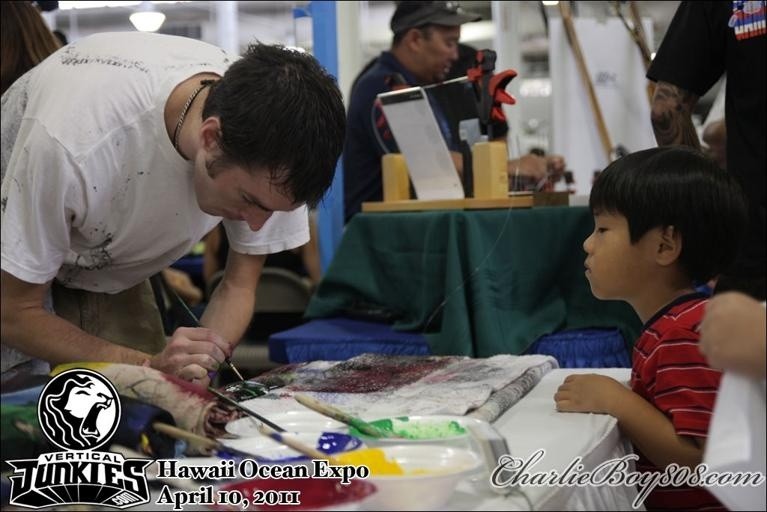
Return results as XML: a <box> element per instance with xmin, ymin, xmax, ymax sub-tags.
<box><xmin>267</xmin><ymin>318</ymin><xmax>632</xmax><ymax>369</ymax></box>
<box><xmin>122</xmin><ymin>360</ymin><xmax>633</xmax><ymax>512</ymax></box>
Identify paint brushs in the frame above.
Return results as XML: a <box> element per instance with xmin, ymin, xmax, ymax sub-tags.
<box><xmin>171</xmin><ymin>288</ymin><xmax>245</xmax><ymax>381</ymax></box>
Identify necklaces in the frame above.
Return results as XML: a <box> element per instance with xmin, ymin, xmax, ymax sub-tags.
<box><xmin>173</xmin><ymin>77</ymin><xmax>214</xmax><ymax>161</ymax></box>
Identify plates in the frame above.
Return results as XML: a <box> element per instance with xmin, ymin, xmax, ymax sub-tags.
<box><xmin>224</xmin><ymin>411</ymin><xmax>349</xmax><ymax>435</ymax></box>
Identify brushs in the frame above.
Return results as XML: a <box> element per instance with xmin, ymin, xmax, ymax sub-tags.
<box><xmin>152</xmin><ymin>387</ymin><xmax>408</xmax><ymax>482</ymax></box>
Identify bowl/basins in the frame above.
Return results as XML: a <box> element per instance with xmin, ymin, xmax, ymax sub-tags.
<box><xmin>205</xmin><ymin>414</ymin><xmax>491</xmax><ymax>512</ymax></box>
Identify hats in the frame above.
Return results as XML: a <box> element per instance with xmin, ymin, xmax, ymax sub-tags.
<box><xmin>390</xmin><ymin>2</ymin><xmax>480</xmax><ymax>34</ymax></box>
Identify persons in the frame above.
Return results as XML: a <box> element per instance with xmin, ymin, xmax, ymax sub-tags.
<box><xmin>551</xmin><ymin>142</ymin><xmax>751</xmax><ymax>511</ymax></box>
<box><xmin>340</xmin><ymin>2</ymin><xmax>568</xmax><ymax>230</ymax></box>
<box><xmin>0</xmin><ymin>0</ymin><xmax>63</xmax><ymax>102</ymax></box>
<box><xmin>696</xmin><ymin>290</ymin><xmax>766</xmax><ymax>384</ymax></box>
<box><xmin>0</xmin><ymin>28</ymin><xmax>348</xmax><ymax>390</ymax></box>
<box><xmin>430</xmin><ymin>44</ymin><xmax>510</xmax><ymax>158</ymax></box>
<box><xmin>644</xmin><ymin>0</ymin><xmax>766</xmax><ymax>306</ymax></box>
